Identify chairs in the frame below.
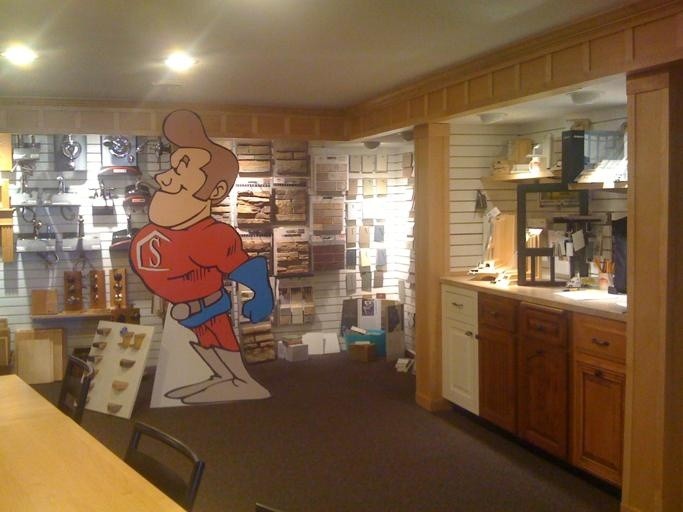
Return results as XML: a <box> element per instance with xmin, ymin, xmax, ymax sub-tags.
<box><xmin>56</xmin><ymin>354</ymin><xmax>95</xmax><ymax>421</ymax></box>
<box><xmin>123</xmin><ymin>421</ymin><xmax>206</xmax><ymax>512</ymax></box>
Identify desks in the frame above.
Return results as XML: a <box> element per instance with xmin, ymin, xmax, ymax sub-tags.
<box><xmin>0</xmin><ymin>374</ymin><xmax>196</xmax><ymax>512</ymax></box>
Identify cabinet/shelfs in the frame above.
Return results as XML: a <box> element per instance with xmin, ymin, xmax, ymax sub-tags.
<box><xmin>441</xmin><ymin>283</ymin><xmax>627</xmax><ymax>493</ymax></box>
<box><xmin>9</xmin><ymin>151</ymin><xmax>103</xmax><ymax>255</ymax></box>
